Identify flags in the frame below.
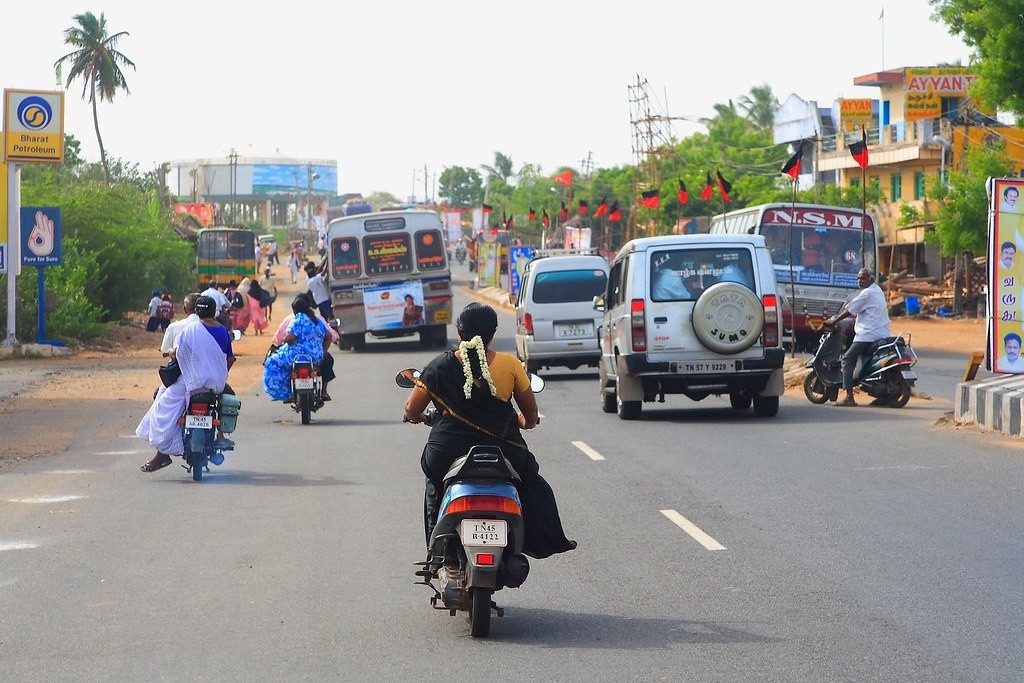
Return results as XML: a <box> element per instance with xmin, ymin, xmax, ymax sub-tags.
<box><xmin>530</xmin><ymin>208</ymin><xmax>549</xmax><ymax>226</ymax></box>
<box><xmin>701</xmin><ymin>174</ymin><xmax>715</xmax><ymax>200</ymax></box>
<box><xmin>427</xmin><ymin>202</ymin><xmax>472</xmax><ymax>214</ymax></box>
<box><xmin>679</xmin><ymin>179</ymin><xmax>688</xmax><ymax>204</ymax></box>
<box><xmin>559</xmin><ymin>200</ymin><xmax>588</xmax><ymax>218</ymax></box>
<box><xmin>781</xmin><ymin>147</ymin><xmax>803</xmax><ymax>179</ymax></box>
<box><xmin>594</xmin><ymin>197</ymin><xmax>621</xmax><ymax>222</ymax></box>
<box><xmin>637</xmin><ymin>189</ymin><xmax>659</xmax><ymax>209</ymax></box>
<box><xmin>491</xmin><ymin>210</ymin><xmax>513</xmax><ymax>234</ymax></box>
<box><xmin>482</xmin><ymin>204</ymin><xmax>493</xmax><ymax>213</ymax></box>
<box><xmin>848</xmin><ymin>131</ymin><xmax>868</xmax><ymax>169</ymax></box>
<box><xmin>716</xmin><ymin>170</ymin><xmax>732</xmax><ymax>202</ymax></box>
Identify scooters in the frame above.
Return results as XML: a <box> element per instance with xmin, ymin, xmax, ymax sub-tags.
<box><xmin>176</xmin><ymin>329</ymin><xmax>243</xmax><ymax>481</ymax></box>
<box><xmin>803</xmin><ymin>291</ymin><xmax>918</xmax><ymax>408</ymax></box>
<box><xmin>393</xmin><ymin>367</ymin><xmax>547</xmax><ymax>639</ymax></box>
<box><xmin>283</xmin><ymin>317</ymin><xmax>341</xmax><ymax>426</ymax></box>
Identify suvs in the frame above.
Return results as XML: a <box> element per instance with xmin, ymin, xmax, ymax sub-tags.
<box><xmin>592</xmin><ymin>232</ymin><xmax>789</xmax><ymax>420</ymax></box>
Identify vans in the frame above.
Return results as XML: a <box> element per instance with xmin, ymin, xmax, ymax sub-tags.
<box><xmin>509</xmin><ymin>248</ymin><xmax>612</xmax><ymax>376</ymax></box>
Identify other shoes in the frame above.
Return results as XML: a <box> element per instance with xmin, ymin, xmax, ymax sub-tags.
<box><xmin>832</xmin><ymin>399</ymin><xmax>858</xmax><ymax>407</ymax></box>
<box><xmin>322</xmin><ymin>395</ymin><xmax>331</xmax><ymax>401</ymax></box>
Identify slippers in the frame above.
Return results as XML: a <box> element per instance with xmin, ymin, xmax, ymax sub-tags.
<box><xmin>140</xmin><ymin>459</ymin><xmax>172</xmax><ymax>472</ymax></box>
<box><xmin>215</xmin><ymin>438</ymin><xmax>235</xmax><ymax>445</ymax></box>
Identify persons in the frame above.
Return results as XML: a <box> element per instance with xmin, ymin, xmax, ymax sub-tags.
<box><xmin>266</xmin><ymin>239</ymin><xmax>280</xmax><ymax>264</ymax></box>
<box><xmin>255</xmin><ymin>243</ymin><xmax>262</xmax><ymax>274</ymax></box>
<box><xmin>303</xmin><ymin>252</ymin><xmax>334</xmax><ymax>322</ymax></box>
<box><xmin>134</xmin><ymin>292</ymin><xmax>237</xmax><ymax>472</ymax></box>
<box><xmin>651</xmin><ymin>253</ymin><xmax>694</xmax><ymax>301</ymax></box>
<box><xmin>1002</xmin><ymin>187</ymin><xmax>1020</xmax><ymax>210</ymax></box>
<box><xmin>823</xmin><ymin>267</ymin><xmax>892</xmax><ymax>405</ymax></box>
<box><xmin>404</xmin><ymin>302</ymin><xmax>540</xmax><ymax>543</ymax></box>
<box><xmin>146</xmin><ymin>291</ymin><xmax>161</xmax><ymax>331</ymax></box>
<box><xmin>259</xmin><ymin>266</ymin><xmax>277</xmax><ymax>321</ymax></box>
<box><xmin>397</xmin><ymin>369</ymin><xmax>418</xmax><ymax>385</ymax></box>
<box><xmin>711</xmin><ymin>251</ymin><xmax>750</xmax><ymax>286</ymax></box>
<box><xmin>998</xmin><ymin>242</ymin><xmax>1017</xmax><ymax>271</ymax></box>
<box><xmin>287</xmin><ymin>250</ymin><xmax>301</xmax><ymax>284</ymax></box>
<box><xmin>402</xmin><ymin>295</ymin><xmax>423</xmax><ymax>326</ymax></box>
<box><xmin>998</xmin><ymin>333</ymin><xmax>1024</xmax><ymax>370</ymax></box>
<box><xmin>264</xmin><ymin>293</ymin><xmax>339</xmax><ymax>404</ymax></box>
<box><xmin>200</xmin><ymin>277</ymin><xmax>270</xmax><ymax>336</ymax></box>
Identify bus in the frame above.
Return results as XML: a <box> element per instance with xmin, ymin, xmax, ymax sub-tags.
<box><xmin>708</xmin><ymin>202</ymin><xmax>880</xmax><ymax>350</ymax></box>
<box><xmin>325</xmin><ymin>200</ymin><xmax>455</xmax><ymax>351</ymax></box>
<box><xmin>195</xmin><ymin>225</ymin><xmax>256</xmax><ymax>292</ymax></box>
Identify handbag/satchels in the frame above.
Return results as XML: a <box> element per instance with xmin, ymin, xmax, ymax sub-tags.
<box><xmin>159</xmin><ymin>360</ymin><xmax>181</xmax><ymax>388</ymax></box>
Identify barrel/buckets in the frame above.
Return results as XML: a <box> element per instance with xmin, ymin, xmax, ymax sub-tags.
<box><xmin>906</xmin><ymin>296</ymin><xmax>920</xmax><ymax>315</ymax></box>
